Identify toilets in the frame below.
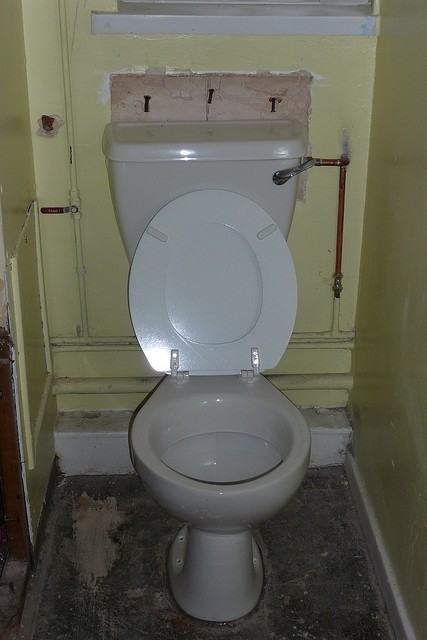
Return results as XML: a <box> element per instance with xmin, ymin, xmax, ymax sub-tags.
<box><xmin>102</xmin><ymin>119</ymin><xmax>312</xmax><ymax>621</ymax></box>
